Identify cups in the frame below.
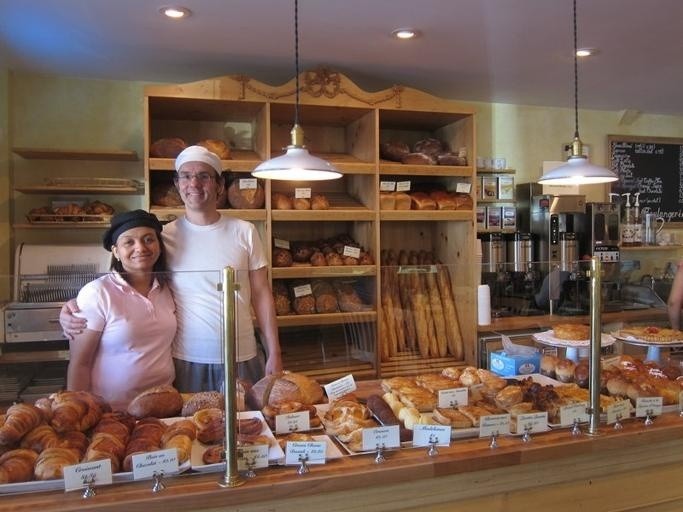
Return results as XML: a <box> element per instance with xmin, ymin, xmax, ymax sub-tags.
<box><xmin>646</xmin><ymin>213</ymin><xmax>665</xmax><ymax>246</ymax></box>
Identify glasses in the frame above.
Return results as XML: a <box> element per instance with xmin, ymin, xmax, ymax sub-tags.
<box><xmin>175</xmin><ymin>174</ymin><xmax>219</xmax><ymax>184</ymax></box>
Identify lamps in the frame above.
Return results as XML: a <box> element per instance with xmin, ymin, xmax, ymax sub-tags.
<box><xmin>158</xmin><ymin>6</ymin><xmax>191</xmax><ymax>22</ymax></box>
<box><xmin>573</xmin><ymin>47</ymin><xmax>597</xmax><ymax>59</ymax></box>
<box><xmin>538</xmin><ymin>0</ymin><xmax>620</xmax><ymax>186</ymax></box>
<box><xmin>391</xmin><ymin>27</ymin><xmax>421</xmax><ymax>41</ymax></box>
<box><xmin>251</xmin><ymin>1</ymin><xmax>344</xmax><ymax>182</ymax></box>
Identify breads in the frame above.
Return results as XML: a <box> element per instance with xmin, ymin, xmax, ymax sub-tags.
<box><xmin>418</xmin><ymin>250</ymin><xmax>439</xmax><ymax>358</ymax></box>
<box><xmin>409</xmin><ymin>250</ymin><xmax>429</xmax><ymax>358</ymax></box>
<box><xmin>279</xmin><ymin>432</ymin><xmax>315</xmax><ymax>452</ymax></box>
<box><xmin>398</xmin><ymin>249</ymin><xmax>417</xmax><ymax>355</ymax></box>
<box><xmin>435</xmin><ymin>260</ymin><xmax>464</xmax><ymax>361</ymax></box>
<box><xmin>325</xmin><ymin>252</ymin><xmax>343</xmax><ymax>266</ymax></box>
<box><xmin>310</xmin><ymin>251</ymin><xmax>327</xmax><ymax>266</ymax></box>
<box><xmin>424</xmin><ymin>259</ymin><xmax>448</xmax><ymax>358</ymax></box>
<box><xmin>123</xmin><ymin>416</ymin><xmax>168</xmax><ymax>473</ymax></box>
<box><xmin>379</xmin><ymin>141</ymin><xmax>411</xmax><ymax>162</ymax></box>
<box><xmin>451</xmin><ymin>193</ymin><xmax>473</xmax><ymax>210</ymax></box>
<box><xmin>324</xmin><ymin>392</ymin><xmax>380</xmax><ymax>452</ymax></box>
<box><xmin>196</xmin><ymin>416</ymin><xmax>262</xmax><ymax>446</ymax></box>
<box><xmin>271</xmin><ymin>192</ymin><xmax>292</xmax><ymax>210</ymax></box>
<box><xmin>30</xmin><ymin>206</ymin><xmax>55</xmax><ymax>220</ymax></box>
<box><xmin>261</xmin><ymin>396</ymin><xmax>321</xmax><ymax>420</ymax></box>
<box><xmin>317</xmin><ymin>233</ymin><xmax>365</xmax><ymax>254</ymax></box>
<box><xmin>82</xmin><ymin>200</ymin><xmax>115</xmax><ymax>215</ymax></box>
<box><xmin>553</xmin><ymin>322</ymin><xmax>591</xmax><ymax>342</ymax></box>
<box><xmin>429</xmin><ymin>191</ymin><xmax>456</xmax><ymax>210</ymax></box>
<box><xmin>34</xmin><ymin>447</ymin><xmax>80</xmax><ymax>481</ymax></box>
<box><xmin>388</xmin><ymin>248</ymin><xmax>406</xmax><ymax>352</ymax></box>
<box><xmin>538</xmin><ymin>354</ymin><xmax>683</xmax><ymax>405</ymax></box>
<box><xmin>253</xmin><ymin>369</ymin><xmax>324</xmax><ymax>410</ymax></box>
<box><xmin>380</xmin><ymin>249</ymin><xmax>398</xmax><ymax>357</ymax></box>
<box><xmin>195</xmin><ymin>137</ymin><xmax>232</xmax><ymax>160</ymax></box>
<box><xmin>331</xmin><ymin>279</ymin><xmax>363</xmax><ymax>312</ymax></box>
<box><xmin>290</xmin><ymin>243</ymin><xmax>320</xmax><ymax>263</ymax></box>
<box><xmin>160</xmin><ymin>419</ymin><xmax>197</xmax><ymax>464</ymax></box>
<box><xmin>149</xmin><ymin>136</ymin><xmax>189</xmax><ymax>158</ymax></box>
<box><xmin>127</xmin><ymin>383</ymin><xmax>184</xmax><ymax>420</ymax></box>
<box><xmin>202</xmin><ymin>433</ymin><xmax>272</xmax><ymax>464</ymax></box>
<box><xmin>227</xmin><ymin>177</ymin><xmax>264</xmax><ymax>209</ymax></box>
<box><xmin>401</xmin><ymin>152</ymin><xmax>435</xmax><ymax>164</ymax></box>
<box><xmin>380</xmin><ymin>304</ymin><xmax>390</xmax><ymax>362</ymax></box>
<box><xmin>412</xmin><ymin>191</ymin><xmax>437</xmax><ymax>210</ymax></box>
<box><xmin>311</xmin><ymin>278</ymin><xmax>338</xmax><ymax>314</ymax></box>
<box><xmin>310</xmin><ymin>193</ymin><xmax>330</xmax><ymax>210</ymax></box>
<box><xmin>272</xmin><ymin>280</ymin><xmax>291</xmax><ymax>317</ymax></box>
<box><xmin>81</xmin><ymin>408</ymin><xmax>137</xmax><ymax>474</ymax></box>
<box><xmin>266</xmin><ymin>415</ymin><xmax>321</xmax><ymax>428</ymax></box>
<box><xmin>289</xmin><ymin>279</ymin><xmax>316</xmax><ymax>315</ymax></box>
<box><xmin>413</xmin><ymin>137</ymin><xmax>444</xmax><ymax>160</ymax></box>
<box><xmin>394</xmin><ymin>192</ymin><xmax>411</xmax><ymax>210</ymax></box>
<box><xmin>381</xmin><ymin>365</ymin><xmax>541</xmax><ymax>431</ymax></box>
<box><xmin>219</xmin><ymin>377</ymin><xmax>252</xmax><ymax>412</ymax></box>
<box><xmin>359</xmin><ymin>252</ymin><xmax>374</xmax><ymax>265</ymax></box>
<box><xmin>43</xmin><ymin>430</ymin><xmax>90</xmax><ymax>460</ymax></box>
<box><xmin>215</xmin><ymin>188</ymin><xmax>227</xmax><ymax>209</ymax></box>
<box><xmin>152</xmin><ymin>179</ymin><xmax>184</xmax><ymax>207</ymax></box>
<box><xmin>289</xmin><ymin>194</ymin><xmax>311</xmax><ymax>210</ymax></box>
<box><xmin>380</xmin><ymin>193</ymin><xmax>396</xmax><ymax>210</ymax></box>
<box><xmin>366</xmin><ymin>394</ymin><xmax>414</xmax><ymax>443</ymax></box>
<box><xmin>192</xmin><ymin>406</ymin><xmax>223</xmax><ymax>429</ymax></box>
<box><xmin>55</xmin><ymin>203</ymin><xmax>87</xmax><ymax>215</ymax></box>
<box><xmin>504</xmin><ymin>375</ymin><xmax>622</xmax><ymax>425</ymax></box>
<box><xmin>34</xmin><ymin>389</ymin><xmax>102</xmax><ymax>435</ymax></box>
<box><xmin>181</xmin><ymin>390</ymin><xmax>225</xmax><ymax>417</ymax></box>
<box><xmin>0</xmin><ymin>402</ymin><xmax>44</xmax><ymax>448</ymax></box>
<box><xmin>272</xmin><ymin>247</ymin><xmax>293</xmax><ymax>267</ymax></box>
<box><xmin>340</xmin><ymin>253</ymin><xmax>359</xmax><ymax>265</ymax></box>
<box><xmin>618</xmin><ymin>326</ymin><xmax>683</xmax><ymax>343</ymax></box>
<box><xmin>18</xmin><ymin>420</ymin><xmax>57</xmax><ymax>455</ymax></box>
<box><xmin>437</xmin><ymin>151</ymin><xmax>467</xmax><ymax>166</ymax></box>
<box><xmin>0</xmin><ymin>448</ymin><xmax>39</xmax><ymax>484</ymax></box>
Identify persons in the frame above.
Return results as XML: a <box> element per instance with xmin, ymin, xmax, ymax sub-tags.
<box><xmin>67</xmin><ymin>209</ymin><xmax>177</xmax><ymax>410</ymax></box>
<box><xmin>60</xmin><ymin>145</ymin><xmax>282</xmax><ymax>394</ymax></box>
<box><xmin>668</xmin><ymin>256</ymin><xmax>683</xmax><ymax>332</ymax></box>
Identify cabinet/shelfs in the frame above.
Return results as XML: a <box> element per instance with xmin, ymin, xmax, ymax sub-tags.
<box><xmin>476</xmin><ymin>282</ymin><xmax>672</xmax><ymax>345</ymax></box>
<box><xmin>146</xmin><ymin>69</ymin><xmax>478</xmax><ymax>379</ymax></box>
<box><xmin>620</xmin><ymin>241</ymin><xmax>681</xmax><ymax>251</ymax></box>
<box><xmin>478</xmin><ymin>167</ymin><xmax>515</xmax><ymax>233</ymax></box>
<box><xmin>10</xmin><ymin>134</ymin><xmax>138</xmax><ymax>240</ymax></box>
<box><xmin>0</xmin><ymin>351</ymin><xmax>683</xmax><ymax>511</ymax></box>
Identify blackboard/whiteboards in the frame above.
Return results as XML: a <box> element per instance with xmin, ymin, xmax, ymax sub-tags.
<box><xmin>605</xmin><ymin>133</ymin><xmax>683</xmax><ymax>229</ymax></box>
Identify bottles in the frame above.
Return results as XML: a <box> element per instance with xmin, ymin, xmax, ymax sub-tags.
<box><xmin>621</xmin><ymin>192</ymin><xmax>634</xmax><ymax>247</ymax></box>
<box><xmin>633</xmin><ymin>192</ymin><xmax>643</xmax><ymax>247</ymax></box>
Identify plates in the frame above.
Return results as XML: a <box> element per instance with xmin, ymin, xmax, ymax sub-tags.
<box><xmin>530</xmin><ymin>335</ymin><xmax>616</xmax><ymax>348</ymax></box>
<box><xmin>500</xmin><ymin>373</ymin><xmax>637</xmax><ymax>430</ymax></box>
<box><xmin>271</xmin><ymin>409</ymin><xmax>324</xmax><ymax>433</ymax></box>
<box><xmin>277</xmin><ymin>435</ymin><xmax>343</xmax><ymax>466</ymax></box>
<box><xmin>0</xmin><ymin>417</ymin><xmax>191</xmax><ymax>494</ymax></box>
<box><xmin>24</xmin><ymin>213</ymin><xmax>114</xmax><ymax>224</ymax></box>
<box><xmin>612</xmin><ymin>332</ymin><xmax>683</xmax><ymax>347</ymax></box>
<box><xmin>367</xmin><ymin>397</ymin><xmax>412</xmax><ymax>450</ymax></box>
<box><xmin>381</xmin><ymin>378</ymin><xmax>480</xmax><ymax>438</ymax></box>
<box><xmin>313</xmin><ymin>404</ymin><xmax>393</xmax><ymax>456</ymax></box>
<box><xmin>191</xmin><ymin>411</ymin><xmax>285</xmax><ymax>472</ymax></box>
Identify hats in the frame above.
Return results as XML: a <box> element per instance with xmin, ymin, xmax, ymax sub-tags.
<box><xmin>173</xmin><ymin>144</ymin><xmax>223</xmax><ymax>177</ymax></box>
<box><xmin>102</xmin><ymin>207</ymin><xmax>164</xmax><ymax>251</ymax></box>
